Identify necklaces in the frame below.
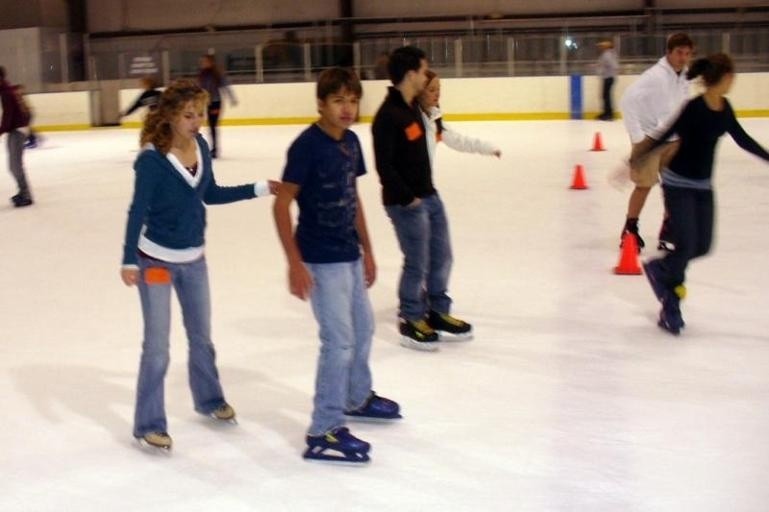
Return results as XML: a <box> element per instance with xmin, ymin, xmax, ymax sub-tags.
<box><xmin>173</xmin><ymin>145</ymin><xmax>197</xmax><ymax>173</ymax></box>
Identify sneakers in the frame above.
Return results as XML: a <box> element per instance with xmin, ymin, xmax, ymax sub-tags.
<box><xmin>399</xmin><ymin>315</ymin><xmax>438</xmax><ymax>342</ymax></box>
<box><xmin>426</xmin><ymin>313</ymin><xmax>471</xmax><ymax>334</ymax></box>
<box><xmin>343</xmin><ymin>394</ymin><xmax>399</xmax><ymax>418</ymax></box>
<box><xmin>662</xmin><ymin>303</ymin><xmax>683</xmax><ymax>334</ymax></box>
<box><xmin>648</xmin><ymin>260</ymin><xmax>667</xmax><ymax>303</ymax></box>
<box><xmin>305</xmin><ymin>428</ymin><xmax>371</xmax><ymax>453</ymax></box>
<box><xmin>205</xmin><ymin>404</ymin><xmax>237</xmax><ymax>419</ymax></box>
<box><xmin>15</xmin><ymin>197</ymin><xmax>32</xmax><ymax>206</ymax></box>
<box><xmin>658</xmin><ymin>231</ymin><xmax>675</xmax><ymax>245</ymax></box>
<box><xmin>135</xmin><ymin>431</ymin><xmax>171</xmax><ymax>448</ymax></box>
<box><xmin>620</xmin><ymin>226</ymin><xmax>644</xmax><ymax>248</ymax></box>
<box><xmin>11</xmin><ymin>191</ymin><xmax>24</xmax><ymax>200</ymax></box>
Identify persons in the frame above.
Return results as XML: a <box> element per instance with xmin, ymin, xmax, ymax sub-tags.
<box><xmin>608</xmin><ymin>54</ymin><xmax>768</xmax><ymax>333</ymax></box>
<box><xmin>622</xmin><ymin>32</ymin><xmax>699</xmax><ymax>252</ymax></box>
<box><xmin>414</xmin><ymin>72</ymin><xmax>503</xmax><ymax>185</ymax></box>
<box><xmin>371</xmin><ymin>47</ymin><xmax>472</xmax><ymax>350</ymax></box>
<box><xmin>1</xmin><ymin>66</ymin><xmax>33</xmax><ymax>206</ymax></box>
<box><xmin>13</xmin><ymin>84</ymin><xmax>39</xmax><ymax>149</ymax></box>
<box><xmin>121</xmin><ymin>81</ymin><xmax>283</xmax><ymax>453</ymax></box>
<box><xmin>274</xmin><ymin>68</ymin><xmax>403</xmax><ymax>462</ymax></box>
<box><xmin>117</xmin><ymin>75</ymin><xmax>162</xmax><ymax>121</ymax></box>
<box><xmin>197</xmin><ymin>54</ymin><xmax>237</xmax><ymax>159</ymax></box>
<box><xmin>594</xmin><ymin>42</ymin><xmax>619</xmax><ymax>118</ymax></box>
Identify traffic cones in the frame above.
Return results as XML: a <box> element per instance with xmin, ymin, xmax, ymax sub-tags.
<box><xmin>590</xmin><ymin>131</ymin><xmax>607</xmax><ymax>151</ymax></box>
<box><xmin>570</xmin><ymin>165</ymin><xmax>588</xmax><ymax>189</ymax></box>
<box><xmin>614</xmin><ymin>230</ymin><xmax>644</xmax><ymax>274</ymax></box>
